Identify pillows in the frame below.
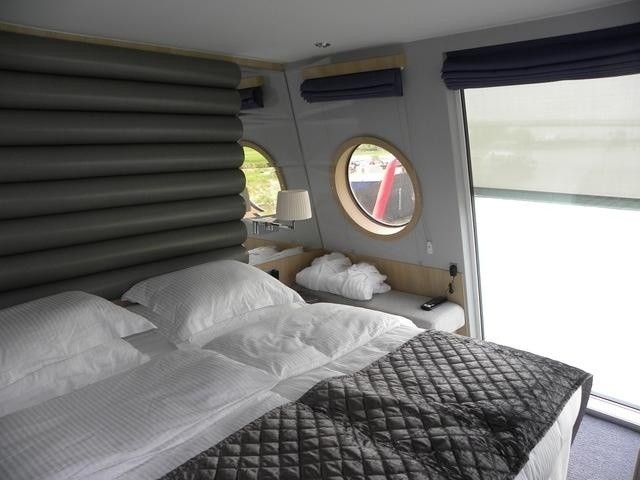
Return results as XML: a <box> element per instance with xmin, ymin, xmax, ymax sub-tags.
<box><xmin>1</xmin><ymin>339</ymin><xmax>151</xmax><ymax>418</ymax></box>
<box><xmin>1</xmin><ymin>290</ymin><xmax>157</xmax><ymax>389</ymax></box>
<box><xmin>121</xmin><ymin>260</ymin><xmax>304</xmax><ymax>337</ymax></box>
<box><xmin>126</xmin><ymin>299</ymin><xmax>308</xmax><ymax>352</ymax></box>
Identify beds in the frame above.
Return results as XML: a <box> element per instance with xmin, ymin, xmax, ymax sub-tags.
<box><xmin>0</xmin><ymin>297</ymin><xmax>584</xmax><ymax>480</ymax></box>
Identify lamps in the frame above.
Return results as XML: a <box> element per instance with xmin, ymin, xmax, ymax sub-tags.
<box><xmin>252</xmin><ymin>190</ymin><xmax>313</xmax><ymax>234</ymax></box>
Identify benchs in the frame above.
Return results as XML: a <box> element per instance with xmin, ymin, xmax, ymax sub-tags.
<box><xmin>295</xmin><ymin>264</ymin><xmax>466</xmax><ymax>334</ymax></box>
